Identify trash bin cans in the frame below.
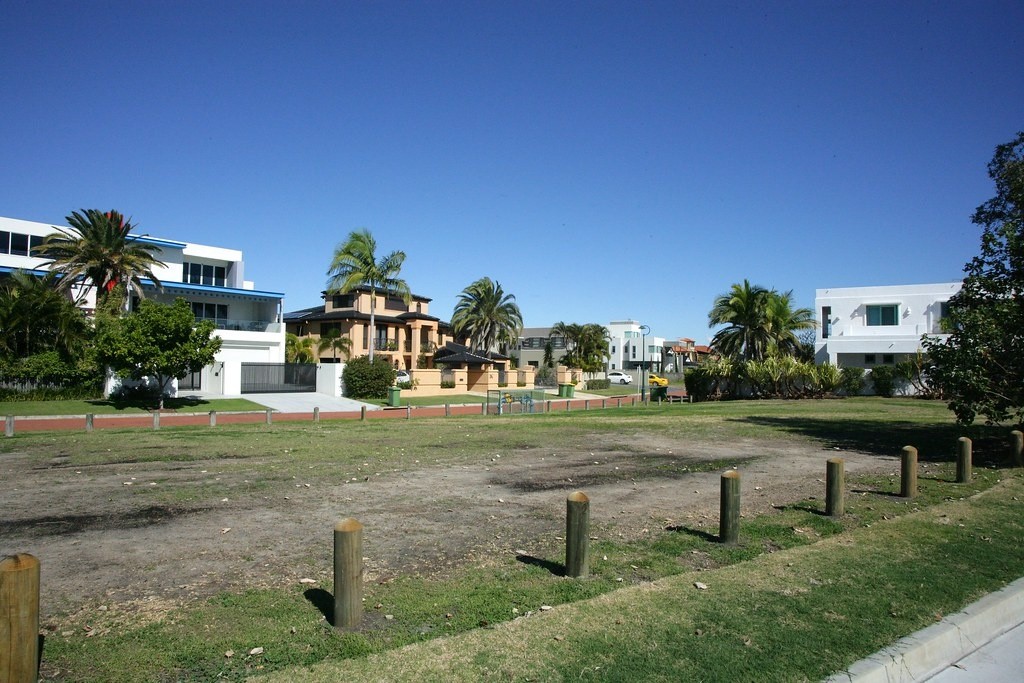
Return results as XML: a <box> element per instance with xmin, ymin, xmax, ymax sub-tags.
<box><xmin>557</xmin><ymin>382</ymin><xmax>575</xmax><ymax>398</ymax></box>
<box><xmin>388</xmin><ymin>387</ymin><xmax>401</xmax><ymax>407</ymax></box>
<box><xmin>649</xmin><ymin>386</ymin><xmax>668</xmax><ymax>402</ymax></box>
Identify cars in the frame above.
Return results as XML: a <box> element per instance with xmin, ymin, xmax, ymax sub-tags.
<box><xmin>397</xmin><ymin>372</ymin><xmax>410</xmax><ymax>384</ymax></box>
<box><xmin>650</xmin><ymin>374</ymin><xmax>668</xmax><ymax>386</ymax></box>
<box><xmin>608</xmin><ymin>372</ymin><xmax>633</xmax><ymax>384</ymax></box>
